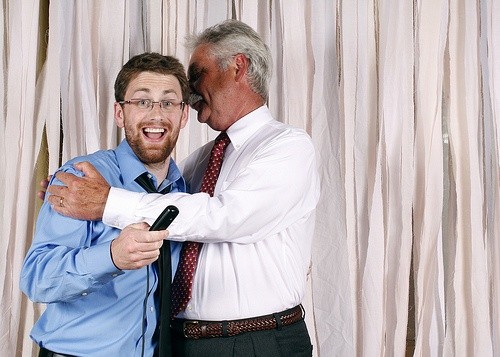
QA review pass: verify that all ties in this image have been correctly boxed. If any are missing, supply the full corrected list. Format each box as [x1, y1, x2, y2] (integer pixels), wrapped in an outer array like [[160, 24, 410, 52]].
[[140, 175, 174, 357], [167, 132, 230, 318]]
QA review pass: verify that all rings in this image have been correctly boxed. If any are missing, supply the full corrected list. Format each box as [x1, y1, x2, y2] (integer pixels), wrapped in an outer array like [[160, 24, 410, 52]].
[[60, 197, 64, 207]]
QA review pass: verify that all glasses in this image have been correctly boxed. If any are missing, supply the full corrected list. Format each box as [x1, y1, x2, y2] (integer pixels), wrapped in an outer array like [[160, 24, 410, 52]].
[[118, 99, 185, 111]]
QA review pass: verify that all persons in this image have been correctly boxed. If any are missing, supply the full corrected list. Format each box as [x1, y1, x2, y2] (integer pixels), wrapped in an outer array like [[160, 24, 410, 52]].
[[38, 20, 320, 357], [19, 52, 189, 357]]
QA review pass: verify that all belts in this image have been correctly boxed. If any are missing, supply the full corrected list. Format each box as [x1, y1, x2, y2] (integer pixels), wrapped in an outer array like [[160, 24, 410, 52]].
[[182, 306, 301, 339], [38, 348, 76, 357]]
